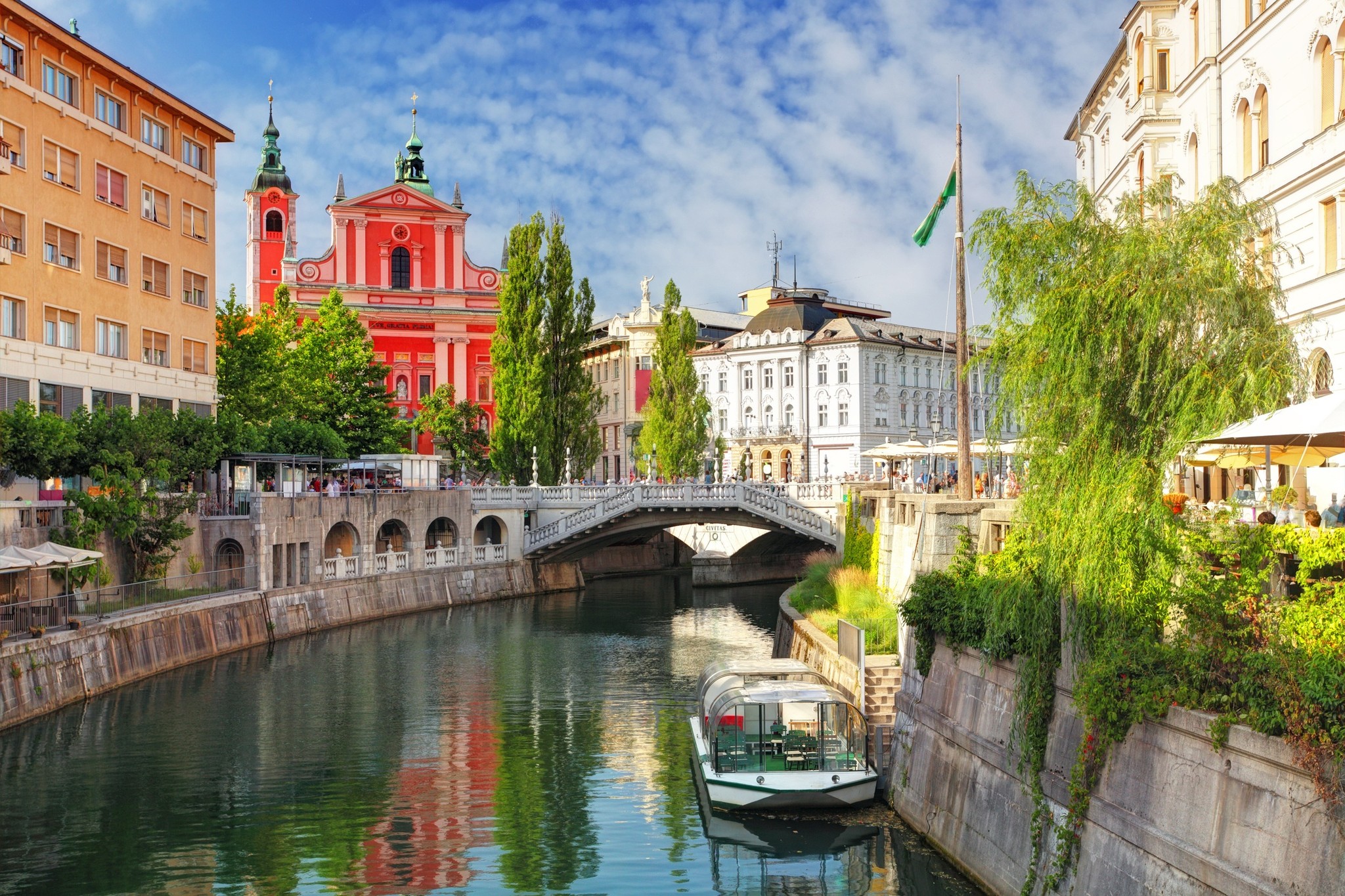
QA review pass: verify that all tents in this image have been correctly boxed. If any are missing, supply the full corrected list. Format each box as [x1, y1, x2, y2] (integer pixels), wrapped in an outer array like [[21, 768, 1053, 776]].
[[1176, 390, 1345, 524], [0, 541, 104, 628], [697, 658, 871, 773]]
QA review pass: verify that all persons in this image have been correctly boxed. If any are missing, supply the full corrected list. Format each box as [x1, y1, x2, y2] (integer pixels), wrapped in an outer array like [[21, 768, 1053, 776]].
[[1336, 506, 1345, 527], [1302, 510, 1321, 528], [892, 471, 897, 490], [916, 470, 958, 494], [266, 478, 275, 492], [440, 476, 484, 490], [704, 470, 714, 484], [1257, 511, 1275, 524], [570, 476, 585, 486], [902, 470, 907, 482], [658, 477, 662, 484], [854, 471, 859, 482], [671, 473, 683, 484], [843, 472, 848, 481], [229, 487, 234, 507], [974, 472, 1021, 500], [640, 477, 646, 485], [691, 476, 696, 484], [307, 472, 402, 497], [730, 468, 739, 481]]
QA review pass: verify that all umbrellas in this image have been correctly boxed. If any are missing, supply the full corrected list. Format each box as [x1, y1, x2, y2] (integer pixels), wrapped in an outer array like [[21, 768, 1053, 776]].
[[859, 436, 1069, 488]]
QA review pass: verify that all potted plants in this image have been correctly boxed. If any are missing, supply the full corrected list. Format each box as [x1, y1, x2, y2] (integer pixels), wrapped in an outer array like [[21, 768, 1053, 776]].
[[30, 627, 47, 639], [69, 618, 82, 629], [0, 630, 9, 647]]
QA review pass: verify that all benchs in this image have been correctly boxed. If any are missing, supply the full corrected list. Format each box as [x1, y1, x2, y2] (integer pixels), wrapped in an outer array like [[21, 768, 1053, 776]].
[[752, 747, 778, 756]]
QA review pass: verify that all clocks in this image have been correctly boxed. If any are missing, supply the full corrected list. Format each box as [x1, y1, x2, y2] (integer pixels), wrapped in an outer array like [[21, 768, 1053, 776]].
[[268, 191, 281, 203], [395, 225, 408, 239]]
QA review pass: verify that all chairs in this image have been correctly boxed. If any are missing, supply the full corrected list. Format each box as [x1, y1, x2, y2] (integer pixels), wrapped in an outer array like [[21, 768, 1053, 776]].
[[712, 724, 752, 773], [770, 722, 858, 771]]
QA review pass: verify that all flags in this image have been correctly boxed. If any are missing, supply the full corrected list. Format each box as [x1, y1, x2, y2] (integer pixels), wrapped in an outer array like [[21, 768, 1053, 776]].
[[912, 157, 956, 249]]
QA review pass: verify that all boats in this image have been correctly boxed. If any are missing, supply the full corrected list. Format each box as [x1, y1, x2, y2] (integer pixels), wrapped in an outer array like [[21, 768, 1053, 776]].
[[690, 655, 878, 814]]
